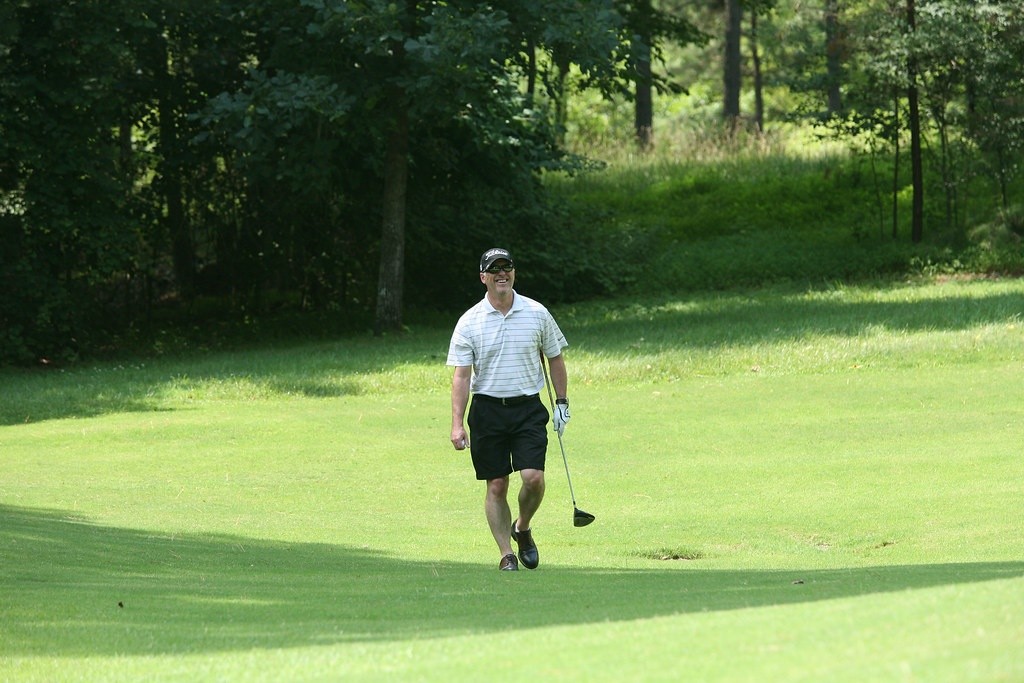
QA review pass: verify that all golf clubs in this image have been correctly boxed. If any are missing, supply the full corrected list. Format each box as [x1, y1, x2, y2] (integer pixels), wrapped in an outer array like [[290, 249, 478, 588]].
[[541, 347, 596, 527]]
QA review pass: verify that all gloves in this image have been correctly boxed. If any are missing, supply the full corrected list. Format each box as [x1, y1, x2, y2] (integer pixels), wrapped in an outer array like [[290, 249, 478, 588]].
[[552, 404, 570, 438]]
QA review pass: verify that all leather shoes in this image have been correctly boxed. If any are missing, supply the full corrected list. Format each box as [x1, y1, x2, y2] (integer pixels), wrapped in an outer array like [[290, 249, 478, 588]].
[[510, 519, 539, 569], [498, 552, 519, 571]]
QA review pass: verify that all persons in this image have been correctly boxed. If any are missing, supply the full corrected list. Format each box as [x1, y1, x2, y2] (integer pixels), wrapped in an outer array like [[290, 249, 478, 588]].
[[446, 248, 569, 571]]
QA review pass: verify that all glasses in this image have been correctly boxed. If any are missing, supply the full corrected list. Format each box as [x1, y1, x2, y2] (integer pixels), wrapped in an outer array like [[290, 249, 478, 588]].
[[485, 263, 515, 274]]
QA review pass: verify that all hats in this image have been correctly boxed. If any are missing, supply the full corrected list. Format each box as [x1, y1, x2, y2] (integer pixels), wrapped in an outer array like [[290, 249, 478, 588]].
[[479, 248, 514, 273]]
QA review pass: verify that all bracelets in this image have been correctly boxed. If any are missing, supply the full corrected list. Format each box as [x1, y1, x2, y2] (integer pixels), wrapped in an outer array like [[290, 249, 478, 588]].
[[555, 398, 569, 405]]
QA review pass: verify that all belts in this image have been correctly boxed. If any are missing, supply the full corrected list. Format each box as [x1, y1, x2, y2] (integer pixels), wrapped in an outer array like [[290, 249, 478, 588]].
[[473, 393, 539, 406]]
